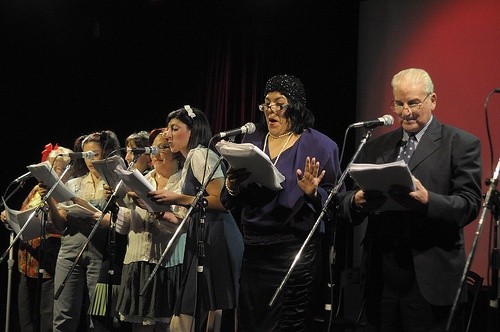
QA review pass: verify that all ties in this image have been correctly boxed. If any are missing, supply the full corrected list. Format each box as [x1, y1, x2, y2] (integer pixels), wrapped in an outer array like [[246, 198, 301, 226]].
[[396, 135, 415, 166]]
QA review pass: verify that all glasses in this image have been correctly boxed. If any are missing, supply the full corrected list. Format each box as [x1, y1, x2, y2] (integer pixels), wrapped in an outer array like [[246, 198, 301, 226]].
[[258, 104, 289, 113], [54, 167, 66, 173], [390, 93, 430, 114]]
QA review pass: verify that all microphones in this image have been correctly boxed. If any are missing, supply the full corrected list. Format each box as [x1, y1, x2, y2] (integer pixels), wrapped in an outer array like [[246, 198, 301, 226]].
[[214, 122, 256, 139], [120, 145, 159, 154], [348, 114, 394, 129], [13, 171, 34, 184], [59, 150, 95, 159]]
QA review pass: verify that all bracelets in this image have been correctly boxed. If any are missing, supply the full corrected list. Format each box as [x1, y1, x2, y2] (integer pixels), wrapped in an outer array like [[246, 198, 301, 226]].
[[155, 211, 165, 219], [225, 177, 238, 196]]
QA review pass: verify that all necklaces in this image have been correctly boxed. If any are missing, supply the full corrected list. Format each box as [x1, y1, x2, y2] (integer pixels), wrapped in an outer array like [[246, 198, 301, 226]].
[[263, 131, 295, 166]]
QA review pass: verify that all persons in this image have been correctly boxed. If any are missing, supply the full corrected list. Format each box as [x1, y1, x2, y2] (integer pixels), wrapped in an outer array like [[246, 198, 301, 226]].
[[221, 74, 346, 332], [38, 130, 121, 332], [0, 147, 73, 332], [335, 69, 481, 332], [152, 105, 244, 332], [88, 127, 187, 332]]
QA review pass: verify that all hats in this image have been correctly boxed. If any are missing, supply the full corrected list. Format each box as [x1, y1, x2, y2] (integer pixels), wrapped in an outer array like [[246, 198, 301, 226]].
[[264, 75, 306, 102]]
